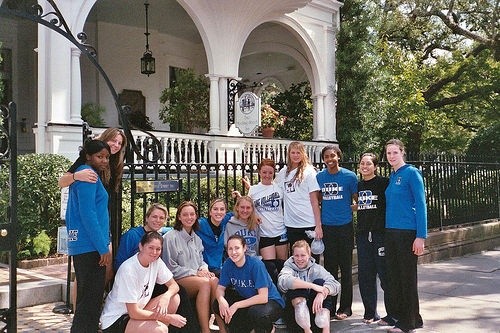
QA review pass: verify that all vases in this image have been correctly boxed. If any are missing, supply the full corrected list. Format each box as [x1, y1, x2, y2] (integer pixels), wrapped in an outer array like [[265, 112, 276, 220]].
[[261, 126, 275, 138]]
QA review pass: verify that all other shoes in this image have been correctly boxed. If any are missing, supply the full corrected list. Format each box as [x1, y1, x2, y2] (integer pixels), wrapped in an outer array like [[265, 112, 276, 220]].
[[387, 327, 416, 333], [378, 320, 389, 326], [336, 313, 347, 320], [364, 318, 374, 323]]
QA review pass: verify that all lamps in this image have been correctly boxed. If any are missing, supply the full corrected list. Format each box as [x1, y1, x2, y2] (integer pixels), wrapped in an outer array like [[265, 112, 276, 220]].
[[141, 3, 155, 77]]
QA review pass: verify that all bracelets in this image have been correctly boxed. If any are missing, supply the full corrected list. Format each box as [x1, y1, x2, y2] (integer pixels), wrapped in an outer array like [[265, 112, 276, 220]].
[[73, 172, 75, 181]]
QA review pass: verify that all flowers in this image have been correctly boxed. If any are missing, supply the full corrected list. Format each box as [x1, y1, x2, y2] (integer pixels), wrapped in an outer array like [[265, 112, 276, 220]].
[[261, 105, 285, 127]]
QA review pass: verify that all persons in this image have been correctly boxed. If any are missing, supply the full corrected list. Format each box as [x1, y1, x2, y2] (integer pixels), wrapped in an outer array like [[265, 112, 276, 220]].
[[98, 139, 429, 333], [58, 128, 128, 324], [66, 139, 113, 333]]
